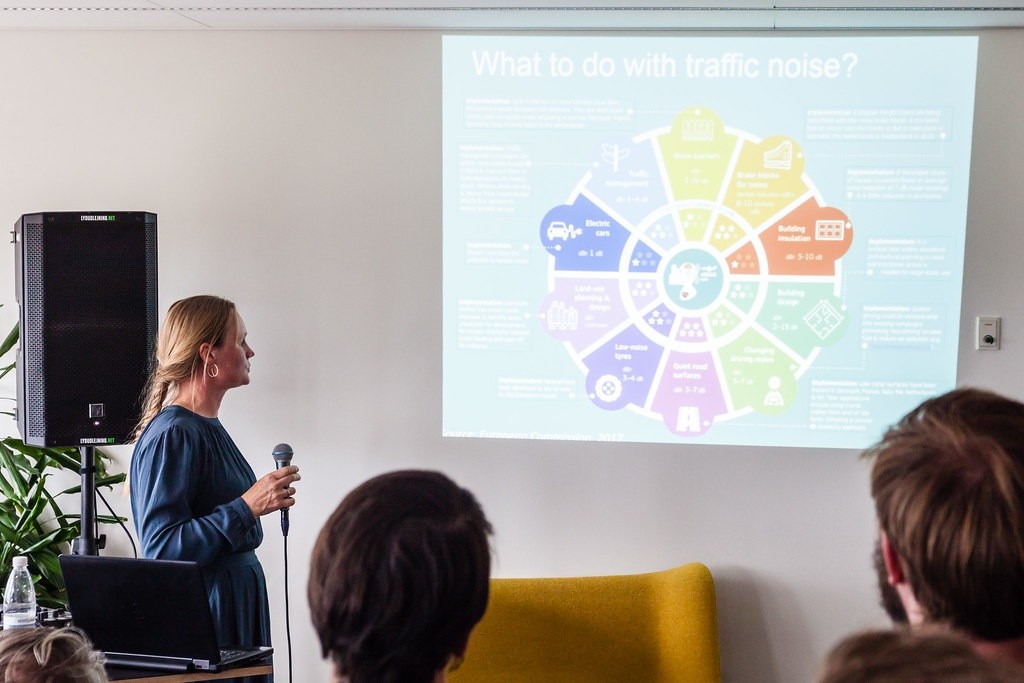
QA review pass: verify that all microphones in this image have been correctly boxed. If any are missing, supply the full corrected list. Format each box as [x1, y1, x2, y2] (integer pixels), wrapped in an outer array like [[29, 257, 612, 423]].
[[272, 443, 294, 537]]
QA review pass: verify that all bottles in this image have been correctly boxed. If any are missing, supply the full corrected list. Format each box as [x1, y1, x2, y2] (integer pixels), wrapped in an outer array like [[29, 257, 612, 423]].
[[3, 557, 37, 629]]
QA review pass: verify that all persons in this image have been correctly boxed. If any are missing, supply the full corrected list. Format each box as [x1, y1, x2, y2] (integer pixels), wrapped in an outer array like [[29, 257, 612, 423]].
[[307, 469, 495, 683], [130, 296, 301, 683], [818, 386, 1024, 682], [0, 628, 107, 683]]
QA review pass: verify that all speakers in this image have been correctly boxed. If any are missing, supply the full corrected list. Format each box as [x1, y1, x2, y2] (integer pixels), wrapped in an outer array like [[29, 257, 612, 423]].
[[10, 211, 160, 448]]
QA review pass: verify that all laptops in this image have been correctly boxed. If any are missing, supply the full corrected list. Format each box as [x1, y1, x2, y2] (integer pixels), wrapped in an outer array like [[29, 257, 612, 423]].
[[59, 554, 275, 673]]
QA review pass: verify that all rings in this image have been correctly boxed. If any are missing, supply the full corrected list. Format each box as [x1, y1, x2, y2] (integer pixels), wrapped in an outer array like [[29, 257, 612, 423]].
[[285, 489, 291, 496]]
